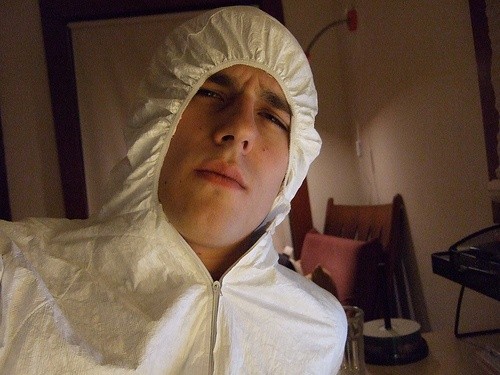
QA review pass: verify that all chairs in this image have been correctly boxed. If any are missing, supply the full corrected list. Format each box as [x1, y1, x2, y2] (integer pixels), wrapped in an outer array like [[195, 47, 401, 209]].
[[279, 193, 403, 325]]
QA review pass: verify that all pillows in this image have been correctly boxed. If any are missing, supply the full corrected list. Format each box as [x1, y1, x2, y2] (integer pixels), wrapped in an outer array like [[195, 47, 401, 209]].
[[279, 227, 381, 315]]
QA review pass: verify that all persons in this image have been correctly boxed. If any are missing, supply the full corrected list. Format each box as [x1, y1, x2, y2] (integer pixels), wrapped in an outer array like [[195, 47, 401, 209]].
[[0, 4, 348, 375]]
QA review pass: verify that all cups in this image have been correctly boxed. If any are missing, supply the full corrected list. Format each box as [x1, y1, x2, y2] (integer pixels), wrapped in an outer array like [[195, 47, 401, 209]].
[[337, 305, 366, 375]]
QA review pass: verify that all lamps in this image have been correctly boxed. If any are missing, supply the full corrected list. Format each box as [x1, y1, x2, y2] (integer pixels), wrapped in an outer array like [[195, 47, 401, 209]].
[[304, 9, 358, 65]]
